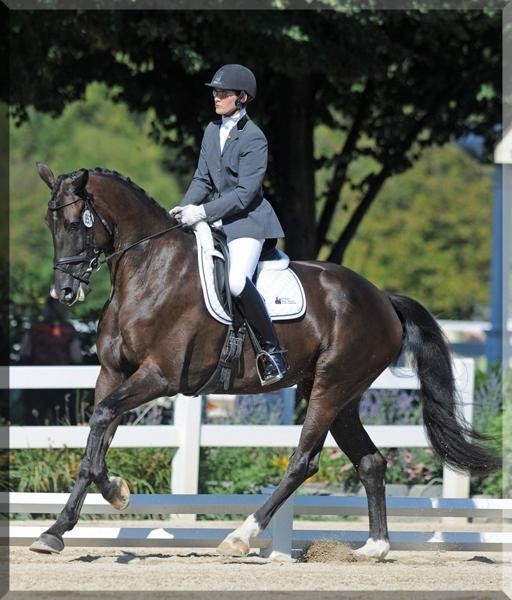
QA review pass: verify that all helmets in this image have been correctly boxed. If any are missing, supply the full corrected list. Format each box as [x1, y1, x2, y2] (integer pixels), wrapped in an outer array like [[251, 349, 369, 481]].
[[205, 65, 257, 97]]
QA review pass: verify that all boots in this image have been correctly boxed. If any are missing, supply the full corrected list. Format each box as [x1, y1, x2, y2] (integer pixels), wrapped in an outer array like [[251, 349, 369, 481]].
[[234, 277, 289, 381]]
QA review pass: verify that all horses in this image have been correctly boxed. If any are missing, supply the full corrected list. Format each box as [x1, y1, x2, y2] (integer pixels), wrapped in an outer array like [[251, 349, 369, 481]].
[[28, 160, 501, 563]]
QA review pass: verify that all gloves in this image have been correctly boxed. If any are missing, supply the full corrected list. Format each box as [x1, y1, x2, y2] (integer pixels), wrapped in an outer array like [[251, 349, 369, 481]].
[[168, 204, 206, 228]]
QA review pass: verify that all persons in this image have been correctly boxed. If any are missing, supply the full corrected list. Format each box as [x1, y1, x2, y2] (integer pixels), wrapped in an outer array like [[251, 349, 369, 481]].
[[168, 64, 291, 381]]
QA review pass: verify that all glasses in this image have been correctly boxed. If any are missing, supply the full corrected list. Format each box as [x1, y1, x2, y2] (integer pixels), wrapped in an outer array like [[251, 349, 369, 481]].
[[212, 89, 234, 97]]
